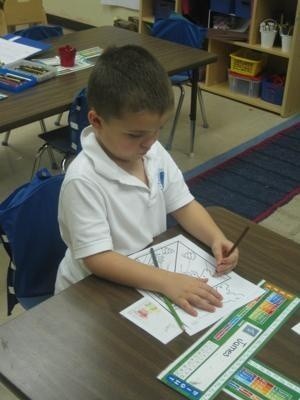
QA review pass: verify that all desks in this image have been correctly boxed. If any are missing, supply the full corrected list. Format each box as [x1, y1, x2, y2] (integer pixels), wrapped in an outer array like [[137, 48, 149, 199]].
[[0, 26, 218, 159], [0, 206, 299, 400]]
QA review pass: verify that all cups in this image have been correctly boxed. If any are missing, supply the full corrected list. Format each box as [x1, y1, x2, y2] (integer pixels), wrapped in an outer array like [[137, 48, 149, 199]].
[[58, 46, 76, 67]]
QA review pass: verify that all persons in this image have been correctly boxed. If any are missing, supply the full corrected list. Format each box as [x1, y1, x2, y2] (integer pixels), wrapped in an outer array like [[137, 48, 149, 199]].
[[53, 42, 241, 316]]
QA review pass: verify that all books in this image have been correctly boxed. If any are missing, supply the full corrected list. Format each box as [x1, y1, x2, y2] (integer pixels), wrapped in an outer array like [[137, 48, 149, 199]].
[[1, 33, 51, 67]]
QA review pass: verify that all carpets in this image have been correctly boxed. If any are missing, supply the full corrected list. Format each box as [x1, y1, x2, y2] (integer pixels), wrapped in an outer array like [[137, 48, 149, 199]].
[[165, 115, 300, 242]]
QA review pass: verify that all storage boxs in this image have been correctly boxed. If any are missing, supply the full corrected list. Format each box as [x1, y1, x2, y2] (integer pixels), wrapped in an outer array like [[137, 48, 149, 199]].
[[228, 69, 261, 98]]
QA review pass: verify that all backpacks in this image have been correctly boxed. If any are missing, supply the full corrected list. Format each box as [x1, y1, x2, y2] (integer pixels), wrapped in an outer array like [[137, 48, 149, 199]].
[[149, 13, 202, 75], [1, 168, 64, 298]]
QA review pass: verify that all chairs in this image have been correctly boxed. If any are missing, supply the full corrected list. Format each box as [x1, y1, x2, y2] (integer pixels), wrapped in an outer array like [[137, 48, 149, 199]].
[[32, 86, 89, 181], [0, 175, 62, 312], [1, 0, 48, 34], [1, 26, 66, 170], [0, 10, 7, 39], [152, 15, 209, 150]]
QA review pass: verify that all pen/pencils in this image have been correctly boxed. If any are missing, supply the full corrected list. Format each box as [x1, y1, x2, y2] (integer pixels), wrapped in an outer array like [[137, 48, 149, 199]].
[[150, 248, 159, 268], [263, 21, 278, 32], [0, 65, 50, 88], [212, 225, 250, 276], [163, 295, 186, 332]]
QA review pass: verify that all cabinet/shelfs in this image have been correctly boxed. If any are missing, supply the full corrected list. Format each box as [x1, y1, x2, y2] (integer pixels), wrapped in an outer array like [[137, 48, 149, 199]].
[[138, 0, 300, 118]]
